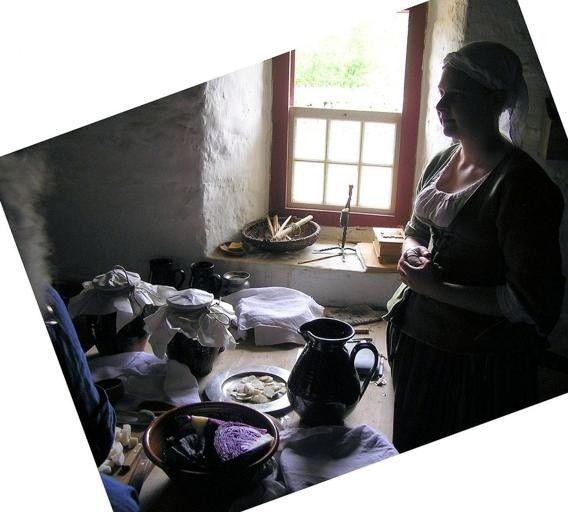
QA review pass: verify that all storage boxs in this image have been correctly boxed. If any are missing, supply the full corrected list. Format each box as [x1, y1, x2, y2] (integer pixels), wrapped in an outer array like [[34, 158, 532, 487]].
[[370, 224, 405, 262]]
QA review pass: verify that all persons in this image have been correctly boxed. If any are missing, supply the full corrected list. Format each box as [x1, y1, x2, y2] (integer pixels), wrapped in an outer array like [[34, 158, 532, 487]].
[[381, 41, 568, 453]]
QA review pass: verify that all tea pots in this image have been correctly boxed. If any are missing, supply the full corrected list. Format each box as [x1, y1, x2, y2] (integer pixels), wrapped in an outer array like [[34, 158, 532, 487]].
[[148, 255, 185, 289], [285, 317, 379, 426], [187, 259, 222, 297]]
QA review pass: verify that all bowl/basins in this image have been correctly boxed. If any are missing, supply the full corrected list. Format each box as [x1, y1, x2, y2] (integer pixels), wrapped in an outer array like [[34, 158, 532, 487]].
[[92, 378, 125, 404], [141, 402, 280, 494]]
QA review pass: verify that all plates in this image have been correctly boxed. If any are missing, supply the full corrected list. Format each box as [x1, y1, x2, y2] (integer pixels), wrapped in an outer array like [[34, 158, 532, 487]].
[[219, 241, 249, 256], [205, 362, 297, 414]]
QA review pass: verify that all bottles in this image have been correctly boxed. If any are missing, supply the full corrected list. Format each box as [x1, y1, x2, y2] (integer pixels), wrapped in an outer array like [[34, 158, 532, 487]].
[[222, 270, 251, 295]]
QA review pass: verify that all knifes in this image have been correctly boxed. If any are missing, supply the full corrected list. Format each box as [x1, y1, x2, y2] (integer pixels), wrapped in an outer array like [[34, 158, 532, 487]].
[[122, 435, 145, 471]]
